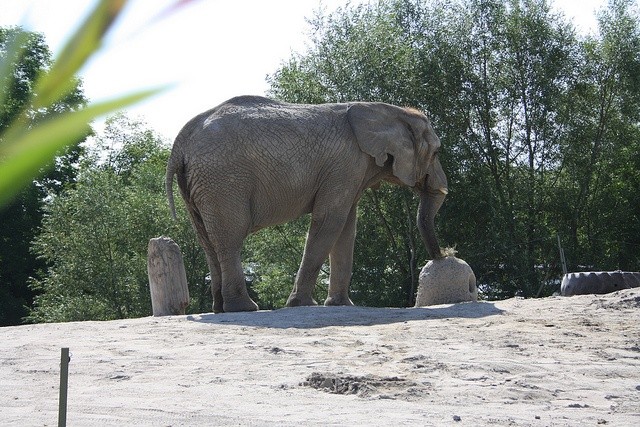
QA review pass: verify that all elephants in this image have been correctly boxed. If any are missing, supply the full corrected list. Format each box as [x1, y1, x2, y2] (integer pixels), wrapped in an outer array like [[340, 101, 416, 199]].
[[166, 96, 448, 313]]
